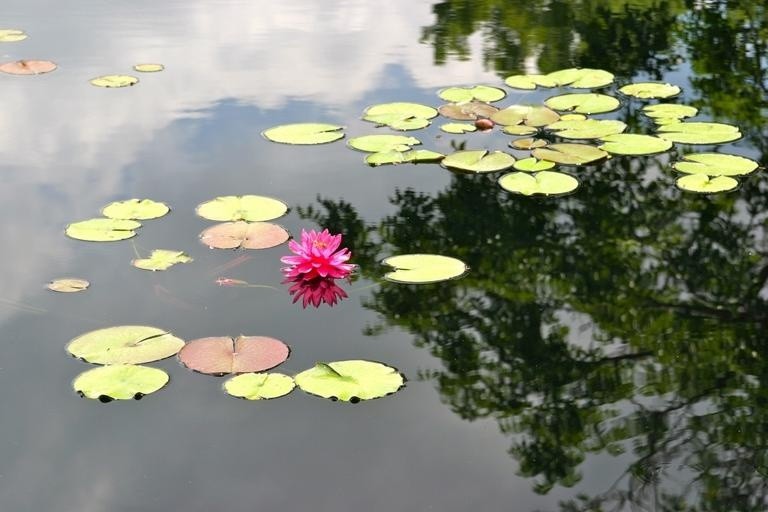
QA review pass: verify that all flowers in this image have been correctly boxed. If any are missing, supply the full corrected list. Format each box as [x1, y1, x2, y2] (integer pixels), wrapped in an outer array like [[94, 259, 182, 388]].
[[280, 228, 358, 282], [288, 283, 349, 309]]
